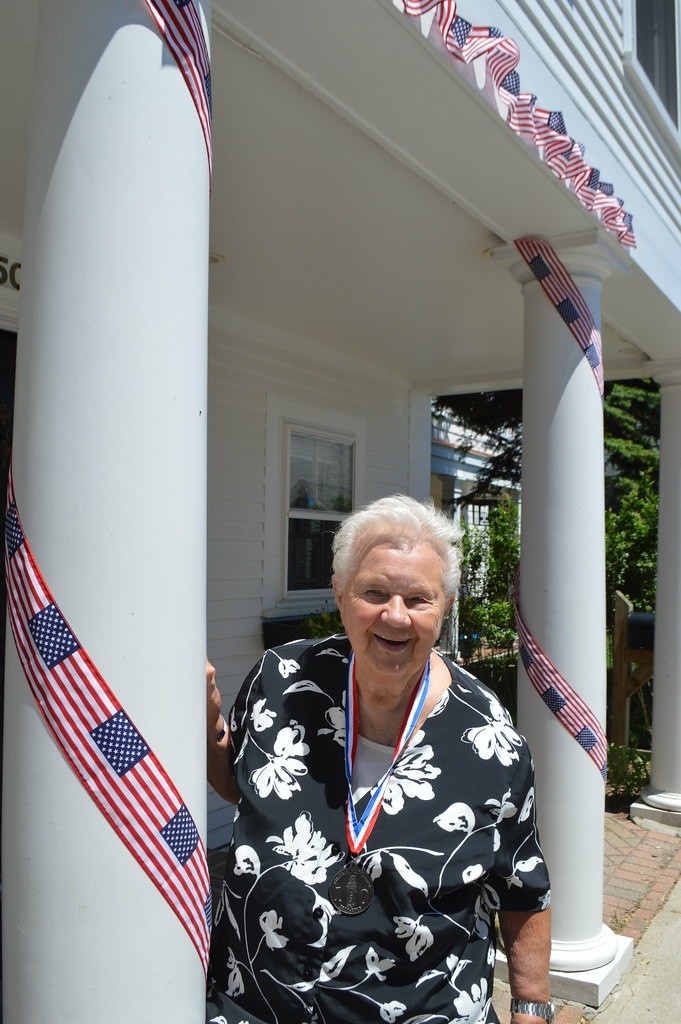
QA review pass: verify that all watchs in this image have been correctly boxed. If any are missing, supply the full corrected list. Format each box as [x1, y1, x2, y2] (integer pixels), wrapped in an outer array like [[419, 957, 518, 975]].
[[510, 998, 555, 1023]]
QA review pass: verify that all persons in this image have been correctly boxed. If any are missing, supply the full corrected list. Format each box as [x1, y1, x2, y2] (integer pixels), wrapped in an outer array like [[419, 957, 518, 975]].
[[206, 493, 554, 1024]]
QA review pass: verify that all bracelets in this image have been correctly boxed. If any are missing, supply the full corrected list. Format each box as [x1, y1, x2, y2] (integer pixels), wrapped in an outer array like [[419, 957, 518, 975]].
[[215, 714, 227, 741]]
